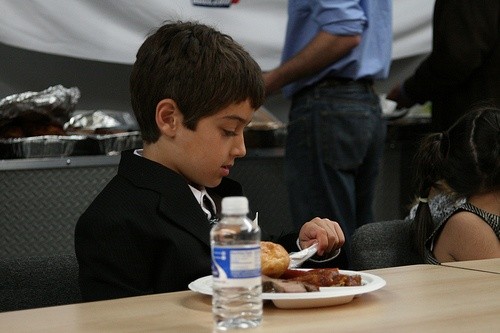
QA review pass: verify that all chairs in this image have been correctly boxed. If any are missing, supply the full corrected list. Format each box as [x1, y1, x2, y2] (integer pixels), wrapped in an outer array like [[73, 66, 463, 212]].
[[0, 249, 80, 312], [350, 217, 418, 270]]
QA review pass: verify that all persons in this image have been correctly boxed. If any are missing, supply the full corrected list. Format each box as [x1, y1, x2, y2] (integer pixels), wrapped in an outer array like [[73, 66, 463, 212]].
[[75, 18, 345, 302], [413, 107, 500, 268], [385, 0, 500, 130], [261, 0, 394, 249]]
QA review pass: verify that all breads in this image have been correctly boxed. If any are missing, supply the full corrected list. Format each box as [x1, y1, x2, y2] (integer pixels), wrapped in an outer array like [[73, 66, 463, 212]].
[[260, 241, 290, 277]]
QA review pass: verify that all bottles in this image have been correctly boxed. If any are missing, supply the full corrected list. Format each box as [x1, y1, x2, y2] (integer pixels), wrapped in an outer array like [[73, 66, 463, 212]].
[[209, 196, 264, 331]]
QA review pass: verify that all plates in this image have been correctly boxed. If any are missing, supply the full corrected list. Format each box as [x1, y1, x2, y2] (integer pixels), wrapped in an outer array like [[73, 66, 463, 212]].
[[188, 268, 387, 310], [384, 109, 409, 125]]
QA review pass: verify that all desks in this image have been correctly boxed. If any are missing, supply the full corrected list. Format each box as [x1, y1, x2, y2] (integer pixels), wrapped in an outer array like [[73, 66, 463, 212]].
[[0, 254, 500, 333]]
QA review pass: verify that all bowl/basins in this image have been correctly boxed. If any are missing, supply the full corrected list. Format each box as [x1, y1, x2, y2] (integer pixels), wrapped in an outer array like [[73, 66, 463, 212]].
[[381, 99, 398, 116]]
